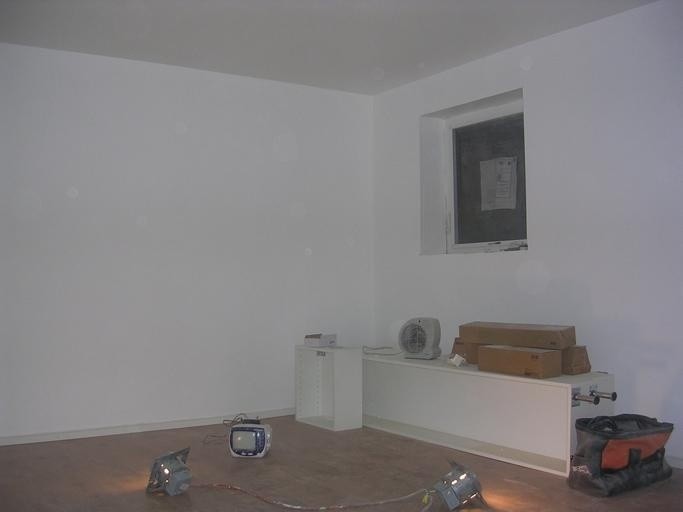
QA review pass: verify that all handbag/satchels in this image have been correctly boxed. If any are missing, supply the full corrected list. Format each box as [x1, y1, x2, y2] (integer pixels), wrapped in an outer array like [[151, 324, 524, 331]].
[[567, 414, 675, 499]]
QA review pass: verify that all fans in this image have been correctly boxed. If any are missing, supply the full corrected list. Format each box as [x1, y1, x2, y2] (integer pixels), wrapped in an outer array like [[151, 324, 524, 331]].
[[394, 315, 442, 361]]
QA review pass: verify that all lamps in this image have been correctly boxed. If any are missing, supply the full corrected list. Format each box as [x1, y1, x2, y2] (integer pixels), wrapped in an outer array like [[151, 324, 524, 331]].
[[144, 445, 196, 497], [427, 457, 491, 511]]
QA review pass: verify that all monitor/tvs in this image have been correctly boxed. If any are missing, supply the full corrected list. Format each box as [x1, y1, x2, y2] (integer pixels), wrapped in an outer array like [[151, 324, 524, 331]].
[[230, 424, 272, 458]]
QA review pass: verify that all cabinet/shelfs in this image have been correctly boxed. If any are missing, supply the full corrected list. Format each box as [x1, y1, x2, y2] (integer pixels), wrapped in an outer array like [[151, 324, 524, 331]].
[[290, 340, 361, 435]]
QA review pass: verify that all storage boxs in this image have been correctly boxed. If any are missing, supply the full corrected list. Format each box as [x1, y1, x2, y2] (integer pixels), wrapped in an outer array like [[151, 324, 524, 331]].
[[447, 319, 592, 381]]
